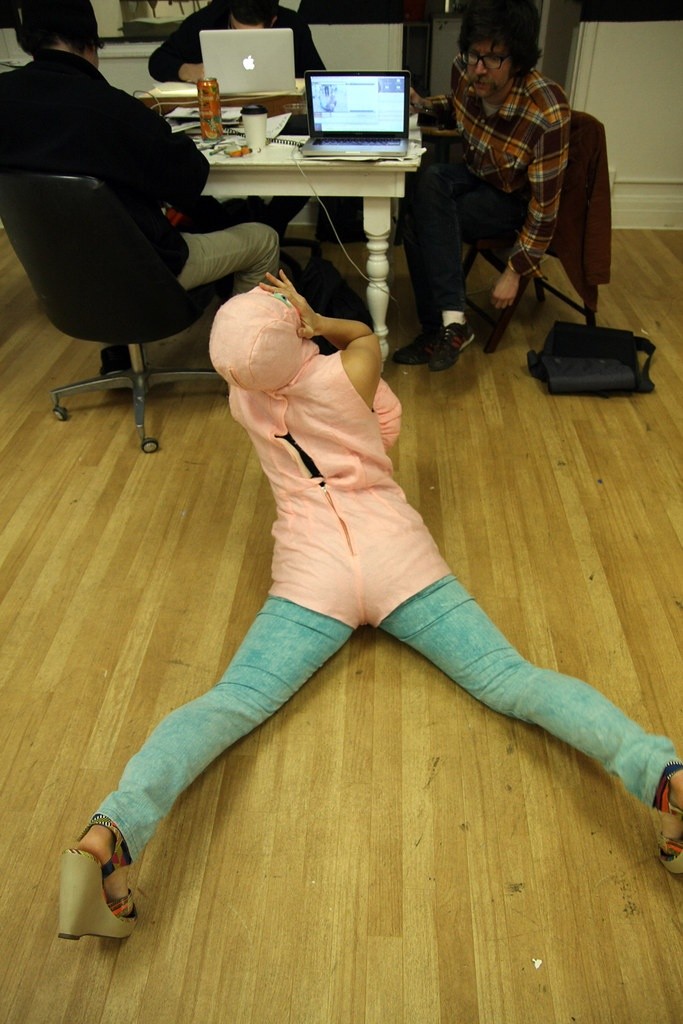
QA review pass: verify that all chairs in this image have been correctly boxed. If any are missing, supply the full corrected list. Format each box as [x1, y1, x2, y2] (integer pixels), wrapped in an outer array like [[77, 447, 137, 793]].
[[453, 110, 609, 355], [0, 175, 230, 453]]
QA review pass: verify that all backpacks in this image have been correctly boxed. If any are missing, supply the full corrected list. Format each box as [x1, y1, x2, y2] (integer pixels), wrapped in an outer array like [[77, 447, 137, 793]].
[[301, 255, 374, 352]]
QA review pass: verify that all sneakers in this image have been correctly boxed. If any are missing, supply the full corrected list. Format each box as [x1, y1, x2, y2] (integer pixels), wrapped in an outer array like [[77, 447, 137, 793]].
[[393, 316, 470, 371]]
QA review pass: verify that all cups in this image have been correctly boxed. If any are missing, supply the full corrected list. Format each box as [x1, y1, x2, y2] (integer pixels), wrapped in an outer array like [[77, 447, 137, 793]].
[[240, 105, 267, 150], [283, 103, 307, 116]]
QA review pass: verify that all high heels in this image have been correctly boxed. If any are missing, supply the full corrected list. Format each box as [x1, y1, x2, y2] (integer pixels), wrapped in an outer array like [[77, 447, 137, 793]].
[[60, 813, 141, 941], [654, 759, 683, 879]]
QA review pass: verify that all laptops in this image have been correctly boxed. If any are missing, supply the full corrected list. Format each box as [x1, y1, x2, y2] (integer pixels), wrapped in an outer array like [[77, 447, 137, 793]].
[[302, 70, 411, 157], [200, 28, 305, 93]]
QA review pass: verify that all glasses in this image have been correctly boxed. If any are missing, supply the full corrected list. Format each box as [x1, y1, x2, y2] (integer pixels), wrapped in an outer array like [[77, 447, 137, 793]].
[[459, 45, 514, 70]]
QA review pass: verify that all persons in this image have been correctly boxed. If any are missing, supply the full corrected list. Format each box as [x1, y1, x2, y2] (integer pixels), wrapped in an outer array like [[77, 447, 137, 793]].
[[0, 0, 279, 297], [148, 0, 326, 241], [391, 0, 571, 371], [57, 272, 683, 942]]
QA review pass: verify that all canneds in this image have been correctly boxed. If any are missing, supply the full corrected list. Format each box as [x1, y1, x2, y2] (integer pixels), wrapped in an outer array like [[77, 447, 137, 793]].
[[197, 78, 223, 140]]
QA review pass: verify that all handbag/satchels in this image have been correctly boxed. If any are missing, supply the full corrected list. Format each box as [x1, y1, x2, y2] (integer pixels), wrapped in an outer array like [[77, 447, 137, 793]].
[[530, 322, 654, 394]]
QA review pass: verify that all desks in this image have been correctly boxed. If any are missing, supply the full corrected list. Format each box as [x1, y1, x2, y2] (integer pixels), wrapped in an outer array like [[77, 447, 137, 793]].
[[136, 111, 424, 375]]
